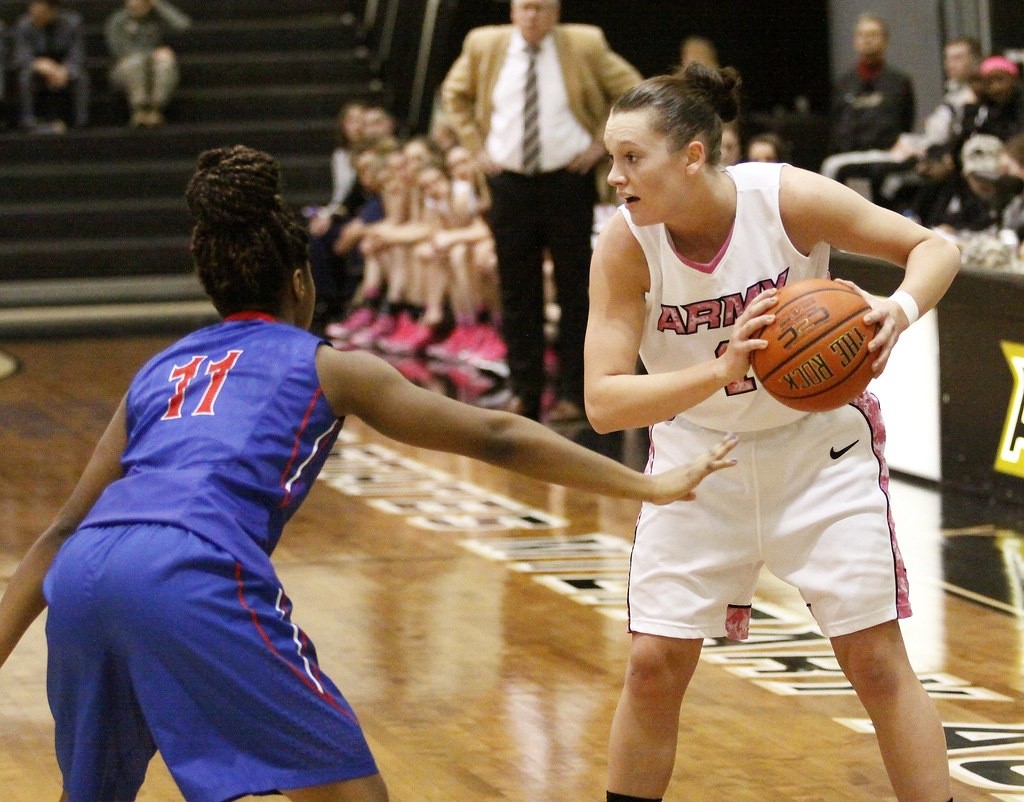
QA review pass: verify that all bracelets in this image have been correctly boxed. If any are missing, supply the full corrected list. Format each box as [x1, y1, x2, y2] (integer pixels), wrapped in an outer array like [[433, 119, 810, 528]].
[[890, 290, 919, 328]]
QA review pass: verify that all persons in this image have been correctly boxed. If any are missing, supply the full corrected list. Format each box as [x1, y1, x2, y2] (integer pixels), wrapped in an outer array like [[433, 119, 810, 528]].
[[10, 0, 93, 133], [103, 0, 192, 127], [582, 62, 961, 802], [0, 144, 740, 802], [307, 105, 624, 382], [442, 0, 647, 416], [682, 16, 1023, 266]]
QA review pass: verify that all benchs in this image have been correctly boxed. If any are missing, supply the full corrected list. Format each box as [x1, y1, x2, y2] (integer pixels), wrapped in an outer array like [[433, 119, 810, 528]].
[[0, 0, 397, 280]]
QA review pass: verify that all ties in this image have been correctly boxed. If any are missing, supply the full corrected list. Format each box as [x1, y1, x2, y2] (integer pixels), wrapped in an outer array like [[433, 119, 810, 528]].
[[521, 46, 542, 175]]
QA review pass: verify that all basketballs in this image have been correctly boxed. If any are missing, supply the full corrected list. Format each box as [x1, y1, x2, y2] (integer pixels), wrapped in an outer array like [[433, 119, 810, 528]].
[[747, 276, 883, 413]]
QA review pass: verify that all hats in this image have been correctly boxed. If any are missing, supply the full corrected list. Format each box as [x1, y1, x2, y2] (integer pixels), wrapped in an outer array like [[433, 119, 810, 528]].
[[961, 134, 1002, 174]]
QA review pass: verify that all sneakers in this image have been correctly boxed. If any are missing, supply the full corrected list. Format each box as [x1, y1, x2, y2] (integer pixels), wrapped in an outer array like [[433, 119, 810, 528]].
[[325, 308, 510, 376]]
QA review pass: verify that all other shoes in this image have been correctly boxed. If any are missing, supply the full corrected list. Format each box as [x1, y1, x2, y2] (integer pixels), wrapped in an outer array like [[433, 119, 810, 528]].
[[512, 395, 540, 423], [132, 110, 162, 126]]
